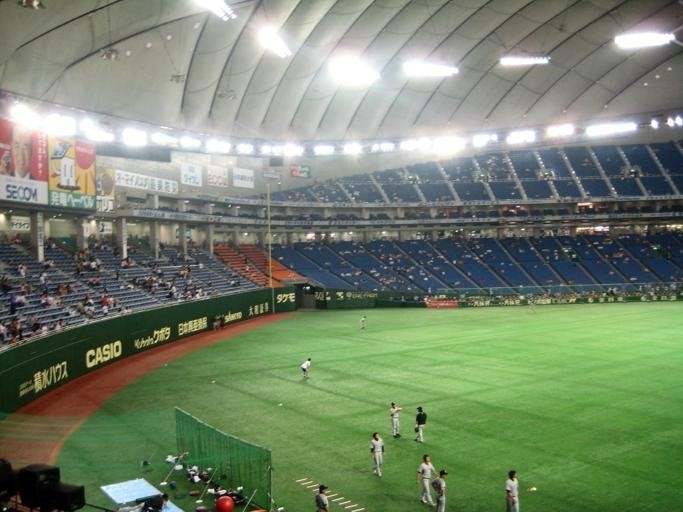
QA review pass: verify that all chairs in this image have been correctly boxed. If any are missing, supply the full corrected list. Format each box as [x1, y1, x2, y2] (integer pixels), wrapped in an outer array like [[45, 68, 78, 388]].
[[268, 233, 681, 304], [404, 143, 680, 229], [2, 236, 267, 345], [118, 194, 266, 220], [269, 167, 422, 204]]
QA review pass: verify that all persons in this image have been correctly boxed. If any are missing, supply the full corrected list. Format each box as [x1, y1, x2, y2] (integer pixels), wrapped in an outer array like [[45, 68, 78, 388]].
[[416, 454, 438, 507], [118, 493, 169, 512], [369, 432, 384, 476], [414, 407, 427, 442], [300, 358, 310, 379], [431, 470, 448, 512], [316, 485, 329, 512], [504, 470, 520, 512], [390, 402, 402, 438]]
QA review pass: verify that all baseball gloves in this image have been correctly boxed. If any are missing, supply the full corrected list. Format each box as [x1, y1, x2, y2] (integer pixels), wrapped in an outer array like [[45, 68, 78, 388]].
[[415, 428, 418, 432]]
